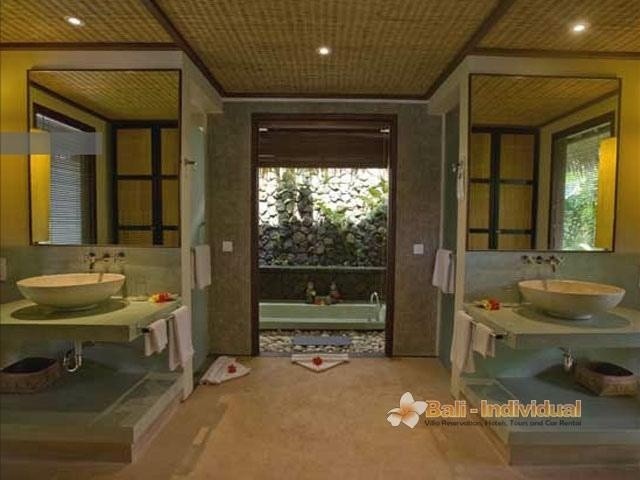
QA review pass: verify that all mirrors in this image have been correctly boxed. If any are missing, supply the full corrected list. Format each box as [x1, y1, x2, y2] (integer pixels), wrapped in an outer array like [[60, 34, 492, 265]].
[[28, 69, 182, 248], [466, 73, 622, 252]]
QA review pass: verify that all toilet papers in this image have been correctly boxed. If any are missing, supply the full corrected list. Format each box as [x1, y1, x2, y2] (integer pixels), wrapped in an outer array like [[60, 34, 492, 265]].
[[329, 283, 340, 302]]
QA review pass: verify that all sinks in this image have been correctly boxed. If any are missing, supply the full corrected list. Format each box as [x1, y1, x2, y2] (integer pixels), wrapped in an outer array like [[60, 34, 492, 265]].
[[16, 273, 125, 311], [517, 279, 627, 320]]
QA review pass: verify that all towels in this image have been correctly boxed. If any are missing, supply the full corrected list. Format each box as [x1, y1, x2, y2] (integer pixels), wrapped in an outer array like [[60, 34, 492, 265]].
[[450, 309, 475, 374], [191, 244, 212, 290], [431, 249, 456, 295], [168, 306, 195, 371], [473, 322, 496, 358], [291, 353, 348, 373], [144, 320, 168, 356], [200, 355, 250, 385]]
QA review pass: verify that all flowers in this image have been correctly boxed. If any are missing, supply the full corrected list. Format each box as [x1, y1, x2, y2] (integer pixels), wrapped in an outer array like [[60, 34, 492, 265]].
[[312, 356, 323, 365], [228, 364, 236, 373], [149, 291, 178, 303]]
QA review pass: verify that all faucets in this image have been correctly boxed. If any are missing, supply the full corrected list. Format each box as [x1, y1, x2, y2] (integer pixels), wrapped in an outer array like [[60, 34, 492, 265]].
[[371, 292, 380, 311], [549, 254, 562, 273], [84, 253, 102, 270]]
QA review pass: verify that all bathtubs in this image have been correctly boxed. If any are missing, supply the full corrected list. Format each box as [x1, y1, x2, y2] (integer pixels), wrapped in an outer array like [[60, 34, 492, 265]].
[[260, 302, 385, 331]]
